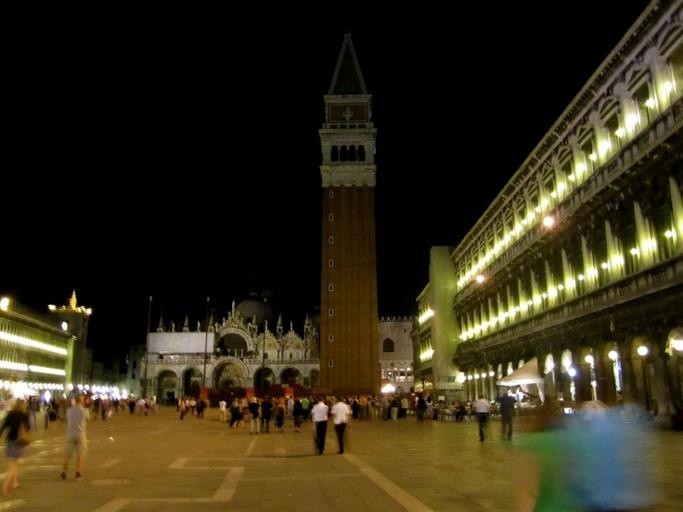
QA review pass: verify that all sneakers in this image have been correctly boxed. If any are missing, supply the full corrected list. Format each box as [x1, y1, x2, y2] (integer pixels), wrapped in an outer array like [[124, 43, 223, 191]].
[[228, 426, 303, 435], [75, 470, 85, 481], [60, 472, 68, 480]]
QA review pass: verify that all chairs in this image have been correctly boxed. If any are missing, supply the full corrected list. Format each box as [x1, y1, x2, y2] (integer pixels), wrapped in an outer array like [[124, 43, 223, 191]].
[[408, 402, 474, 422]]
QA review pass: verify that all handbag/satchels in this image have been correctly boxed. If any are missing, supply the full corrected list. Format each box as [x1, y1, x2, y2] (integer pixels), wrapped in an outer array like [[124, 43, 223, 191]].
[[47, 421, 56, 428], [15, 421, 34, 446]]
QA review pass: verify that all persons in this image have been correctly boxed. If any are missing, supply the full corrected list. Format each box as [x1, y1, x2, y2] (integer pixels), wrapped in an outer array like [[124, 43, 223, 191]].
[[474, 389, 518, 443], [573, 390, 617, 442], [174, 393, 312, 436], [425, 396, 502, 421], [0, 398, 32, 497], [308, 390, 428, 423], [60, 394, 91, 480], [503, 361, 671, 512], [28, 389, 160, 421], [309, 395, 353, 455]]
